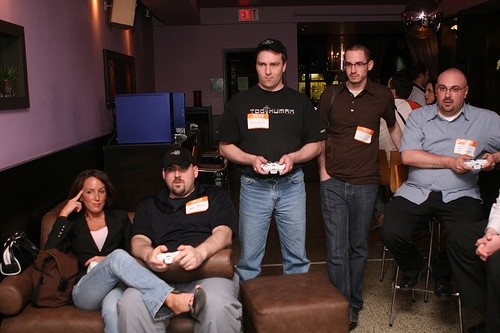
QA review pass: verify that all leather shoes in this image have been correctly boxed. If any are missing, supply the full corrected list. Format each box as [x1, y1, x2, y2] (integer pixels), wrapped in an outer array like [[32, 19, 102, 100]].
[[434, 275, 453, 299], [401, 260, 427, 289]]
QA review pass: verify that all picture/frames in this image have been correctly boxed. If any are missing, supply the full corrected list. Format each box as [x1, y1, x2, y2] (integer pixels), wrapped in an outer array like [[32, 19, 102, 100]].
[[0, 19, 30, 110], [103, 48, 137, 108]]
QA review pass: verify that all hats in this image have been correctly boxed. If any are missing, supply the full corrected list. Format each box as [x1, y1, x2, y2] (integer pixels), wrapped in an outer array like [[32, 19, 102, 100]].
[[162, 146, 193, 171], [256, 38, 288, 60]]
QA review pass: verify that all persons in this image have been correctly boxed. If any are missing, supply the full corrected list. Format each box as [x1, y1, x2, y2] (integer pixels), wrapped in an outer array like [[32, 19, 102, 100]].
[[317, 44, 396, 330], [117, 148, 244, 333], [370, 64, 437, 229], [215, 39, 322, 283], [467, 193, 500, 333], [380, 68, 500, 296], [45, 169, 206, 333]]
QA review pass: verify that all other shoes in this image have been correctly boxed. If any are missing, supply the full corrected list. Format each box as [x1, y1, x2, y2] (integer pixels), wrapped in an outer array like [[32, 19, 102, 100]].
[[369, 214, 385, 230], [467, 322, 487, 333], [375, 240, 387, 247], [348, 305, 359, 332]]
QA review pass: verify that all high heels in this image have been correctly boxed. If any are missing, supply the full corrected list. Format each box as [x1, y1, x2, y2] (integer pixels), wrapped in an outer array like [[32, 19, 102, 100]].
[[175, 287, 207, 323]]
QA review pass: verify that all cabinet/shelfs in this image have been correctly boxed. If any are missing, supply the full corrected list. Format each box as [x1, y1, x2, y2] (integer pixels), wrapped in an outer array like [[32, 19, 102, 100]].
[[103, 106, 212, 213]]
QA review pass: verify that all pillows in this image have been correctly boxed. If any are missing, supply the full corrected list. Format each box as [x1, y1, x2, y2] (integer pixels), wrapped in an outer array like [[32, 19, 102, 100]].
[[133, 247, 234, 283], [0, 263, 36, 316]]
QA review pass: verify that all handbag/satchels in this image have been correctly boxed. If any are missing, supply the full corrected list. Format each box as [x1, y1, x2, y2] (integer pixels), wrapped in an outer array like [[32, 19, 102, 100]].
[[30, 247, 79, 308], [0, 230, 39, 276]]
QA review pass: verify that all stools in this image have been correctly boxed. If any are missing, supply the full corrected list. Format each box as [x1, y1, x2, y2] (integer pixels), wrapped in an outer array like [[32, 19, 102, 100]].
[[377, 148, 464, 333], [240, 270, 350, 333], [196, 142, 229, 189]]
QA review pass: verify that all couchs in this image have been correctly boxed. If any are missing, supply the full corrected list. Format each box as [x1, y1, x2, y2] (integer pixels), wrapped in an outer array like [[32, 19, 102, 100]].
[[0, 199, 235, 333]]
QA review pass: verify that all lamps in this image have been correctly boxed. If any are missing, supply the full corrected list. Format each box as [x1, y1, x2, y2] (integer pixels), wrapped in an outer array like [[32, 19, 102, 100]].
[[403, 0, 442, 39]]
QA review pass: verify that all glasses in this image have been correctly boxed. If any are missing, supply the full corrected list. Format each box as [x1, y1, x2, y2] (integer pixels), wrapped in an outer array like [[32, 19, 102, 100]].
[[437, 86, 467, 93], [344, 60, 371, 67]]
